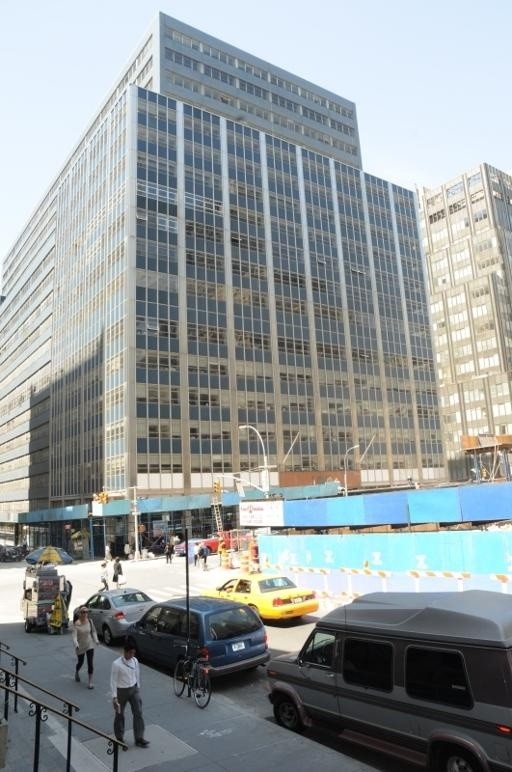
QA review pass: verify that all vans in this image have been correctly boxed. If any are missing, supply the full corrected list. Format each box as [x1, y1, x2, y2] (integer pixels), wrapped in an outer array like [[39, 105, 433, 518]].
[[266, 589, 512, 772]]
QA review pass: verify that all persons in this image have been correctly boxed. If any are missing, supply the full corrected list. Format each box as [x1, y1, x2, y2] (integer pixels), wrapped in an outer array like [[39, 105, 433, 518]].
[[72, 606, 101, 689], [109, 639, 151, 749], [250, 538, 261, 574], [104, 542, 113, 561], [193, 541, 209, 565], [112, 556, 123, 590], [163, 542, 173, 564], [159, 535, 181, 554], [99, 561, 109, 592], [218, 536, 227, 567]]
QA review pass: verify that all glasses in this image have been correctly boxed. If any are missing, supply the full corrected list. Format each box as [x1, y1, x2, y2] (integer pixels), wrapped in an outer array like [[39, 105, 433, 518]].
[[82, 611, 88, 613]]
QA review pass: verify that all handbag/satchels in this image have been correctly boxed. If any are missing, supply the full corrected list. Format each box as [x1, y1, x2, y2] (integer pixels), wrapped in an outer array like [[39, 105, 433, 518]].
[[118, 574, 127, 585]]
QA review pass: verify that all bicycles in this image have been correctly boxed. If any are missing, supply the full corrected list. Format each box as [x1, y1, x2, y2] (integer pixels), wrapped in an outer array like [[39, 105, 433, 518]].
[[171, 639, 214, 710]]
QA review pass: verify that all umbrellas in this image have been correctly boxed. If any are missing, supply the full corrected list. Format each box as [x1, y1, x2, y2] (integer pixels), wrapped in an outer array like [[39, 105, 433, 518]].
[[25, 544, 73, 568], [47, 590, 70, 635]]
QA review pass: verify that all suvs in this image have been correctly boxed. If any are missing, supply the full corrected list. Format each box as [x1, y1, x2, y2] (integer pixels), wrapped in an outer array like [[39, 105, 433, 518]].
[[125, 595, 270, 684]]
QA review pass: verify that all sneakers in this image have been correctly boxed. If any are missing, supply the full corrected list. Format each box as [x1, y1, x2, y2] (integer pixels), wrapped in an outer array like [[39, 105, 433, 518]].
[[89, 685, 94, 689], [75, 673, 80, 682]]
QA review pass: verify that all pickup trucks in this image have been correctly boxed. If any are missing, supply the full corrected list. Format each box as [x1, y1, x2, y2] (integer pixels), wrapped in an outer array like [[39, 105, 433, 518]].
[[194, 531, 249, 558]]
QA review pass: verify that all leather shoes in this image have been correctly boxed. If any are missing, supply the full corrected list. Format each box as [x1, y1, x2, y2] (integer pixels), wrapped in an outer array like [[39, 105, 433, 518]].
[[136, 738, 149, 746]]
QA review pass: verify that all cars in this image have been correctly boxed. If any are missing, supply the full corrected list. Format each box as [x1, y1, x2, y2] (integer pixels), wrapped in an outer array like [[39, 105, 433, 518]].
[[175, 536, 204, 556], [196, 573, 319, 626], [72, 587, 158, 646]]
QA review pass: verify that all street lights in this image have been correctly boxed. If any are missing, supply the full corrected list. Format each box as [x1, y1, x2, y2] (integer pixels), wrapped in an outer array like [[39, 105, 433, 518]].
[[341, 441, 361, 495], [238, 423, 272, 499]]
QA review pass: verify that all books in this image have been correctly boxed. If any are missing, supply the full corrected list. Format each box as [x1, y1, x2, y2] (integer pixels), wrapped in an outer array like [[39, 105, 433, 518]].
[[112, 700, 121, 713]]
